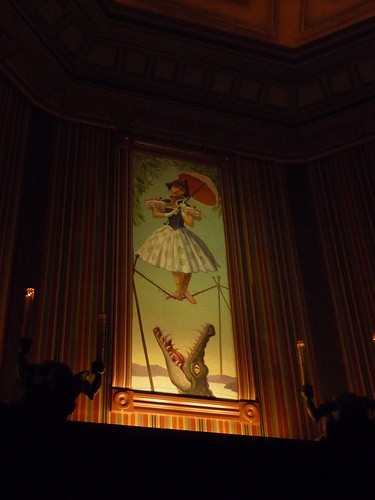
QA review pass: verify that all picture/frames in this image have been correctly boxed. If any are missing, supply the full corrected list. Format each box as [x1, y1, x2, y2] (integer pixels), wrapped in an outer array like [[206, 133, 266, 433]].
[[110, 135, 262, 427]]
